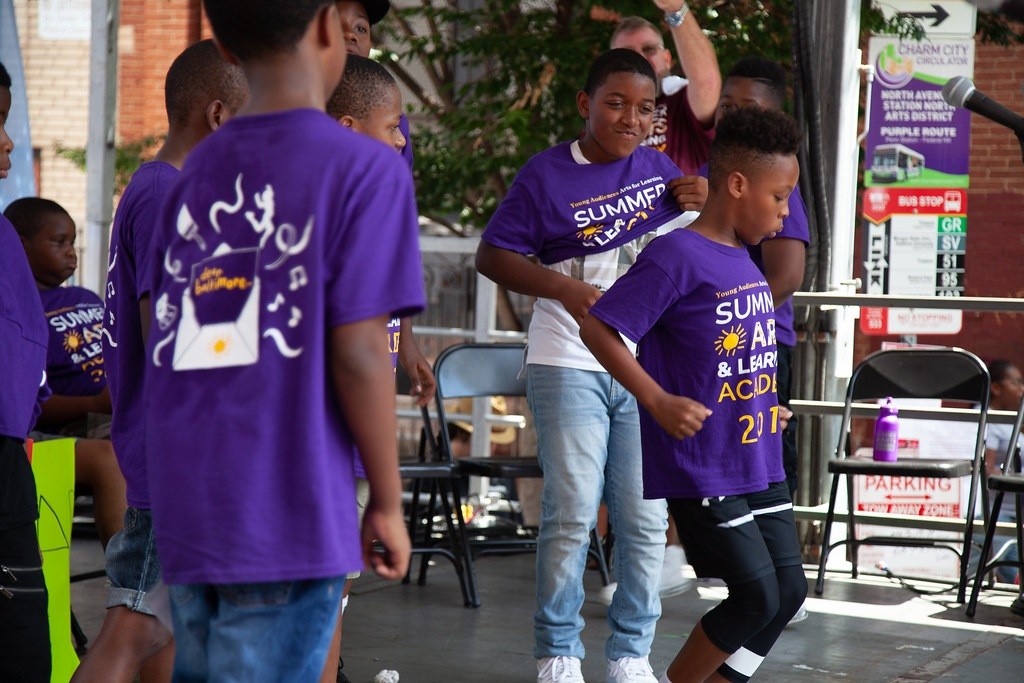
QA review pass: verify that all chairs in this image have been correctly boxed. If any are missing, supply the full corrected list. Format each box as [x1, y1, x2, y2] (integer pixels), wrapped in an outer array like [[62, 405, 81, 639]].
[[355, 362, 472, 608], [815, 347, 997, 605], [418, 342, 611, 608], [966, 392, 1024, 618]]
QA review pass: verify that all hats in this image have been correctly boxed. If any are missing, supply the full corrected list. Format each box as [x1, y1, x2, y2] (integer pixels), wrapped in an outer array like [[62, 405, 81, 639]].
[[356, 0, 390, 27], [451, 395, 516, 445]]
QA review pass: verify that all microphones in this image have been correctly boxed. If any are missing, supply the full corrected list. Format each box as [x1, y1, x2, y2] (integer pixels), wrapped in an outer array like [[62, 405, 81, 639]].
[[942, 76, 1024, 140]]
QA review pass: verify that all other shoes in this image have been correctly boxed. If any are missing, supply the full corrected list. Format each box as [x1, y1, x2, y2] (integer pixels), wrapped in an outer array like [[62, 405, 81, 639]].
[[788, 602, 809, 623], [585, 523, 614, 572]]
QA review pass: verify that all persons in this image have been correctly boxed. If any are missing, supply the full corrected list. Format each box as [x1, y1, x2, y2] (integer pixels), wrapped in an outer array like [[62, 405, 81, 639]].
[[138, 0, 427, 683], [984, 359, 1024, 584], [404, 394, 516, 517], [580, 101, 807, 683], [475, 47, 709, 683], [599, 0, 810, 606], [0, 0, 437, 683]]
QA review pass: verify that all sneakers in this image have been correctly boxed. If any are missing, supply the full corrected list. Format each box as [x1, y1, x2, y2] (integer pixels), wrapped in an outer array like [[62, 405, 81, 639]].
[[536, 655, 585, 683], [605, 654, 658, 682], [598, 545, 697, 604]]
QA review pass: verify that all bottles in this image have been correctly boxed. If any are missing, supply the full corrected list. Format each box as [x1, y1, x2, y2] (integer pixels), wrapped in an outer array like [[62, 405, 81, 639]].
[[872, 396, 899, 462]]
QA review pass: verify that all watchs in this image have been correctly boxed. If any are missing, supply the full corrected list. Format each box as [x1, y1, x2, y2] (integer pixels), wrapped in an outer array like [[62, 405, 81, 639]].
[[664, 1, 689, 26]]
